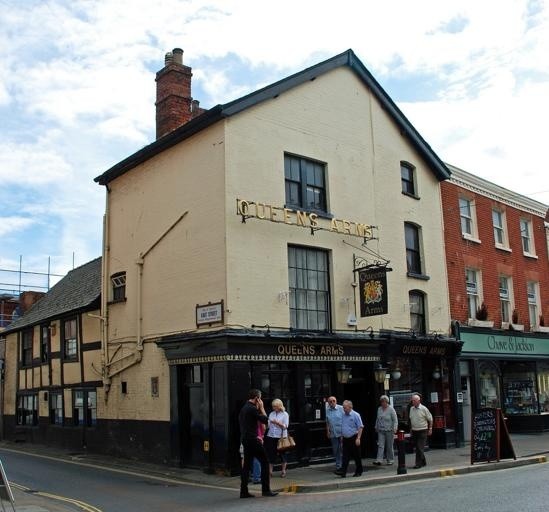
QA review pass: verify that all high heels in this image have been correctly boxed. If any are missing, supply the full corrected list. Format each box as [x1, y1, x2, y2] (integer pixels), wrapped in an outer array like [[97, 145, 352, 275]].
[[281, 470, 287, 478]]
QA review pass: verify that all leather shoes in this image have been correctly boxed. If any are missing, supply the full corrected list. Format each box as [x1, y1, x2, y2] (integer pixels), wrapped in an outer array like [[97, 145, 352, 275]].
[[353, 472, 363, 477], [413, 462, 427, 469], [333, 471, 346, 478], [262, 490, 279, 496], [240, 492, 255, 498]]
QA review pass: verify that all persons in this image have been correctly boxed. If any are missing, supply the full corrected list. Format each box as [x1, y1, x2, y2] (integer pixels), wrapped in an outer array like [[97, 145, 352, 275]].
[[373, 395, 398, 465], [409, 393, 433, 468], [240, 389, 290, 498], [325, 396, 364, 478]]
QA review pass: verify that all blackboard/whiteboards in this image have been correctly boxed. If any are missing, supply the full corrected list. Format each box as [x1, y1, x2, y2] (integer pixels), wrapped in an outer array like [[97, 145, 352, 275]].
[[471, 407, 500, 462]]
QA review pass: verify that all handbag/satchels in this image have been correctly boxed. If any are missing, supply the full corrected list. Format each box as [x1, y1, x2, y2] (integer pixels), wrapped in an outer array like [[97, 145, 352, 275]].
[[277, 428, 296, 450]]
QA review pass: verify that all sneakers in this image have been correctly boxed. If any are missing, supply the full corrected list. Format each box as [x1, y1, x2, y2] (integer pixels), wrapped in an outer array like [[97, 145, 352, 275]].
[[373, 461, 382, 465], [248, 478, 261, 484], [387, 460, 394, 465]]
